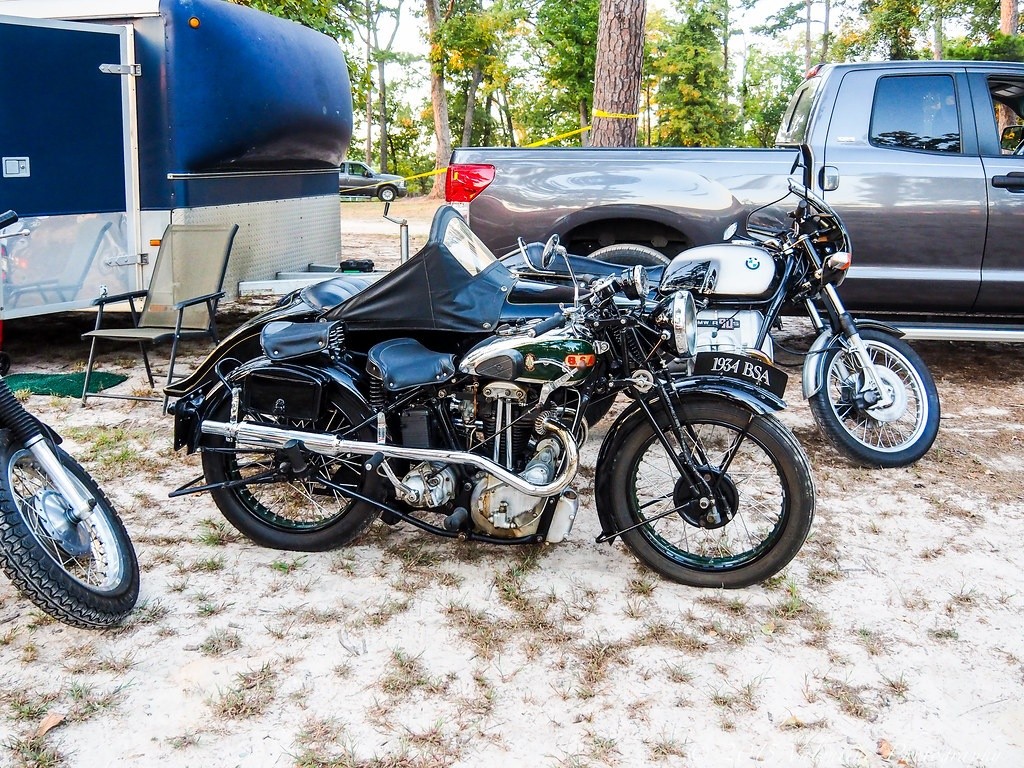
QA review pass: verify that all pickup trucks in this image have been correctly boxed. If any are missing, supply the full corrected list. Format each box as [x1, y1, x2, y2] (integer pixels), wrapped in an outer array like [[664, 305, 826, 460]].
[[448, 46, 1024, 357], [337, 160, 407, 202]]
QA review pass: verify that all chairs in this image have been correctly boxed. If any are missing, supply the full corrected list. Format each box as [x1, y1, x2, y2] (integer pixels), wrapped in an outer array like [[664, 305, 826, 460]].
[[80, 224, 239, 416]]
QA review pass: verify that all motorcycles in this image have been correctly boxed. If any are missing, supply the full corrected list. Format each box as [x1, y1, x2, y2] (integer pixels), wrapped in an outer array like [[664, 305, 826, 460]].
[[437, 146, 943, 476], [168, 222, 821, 595]]
